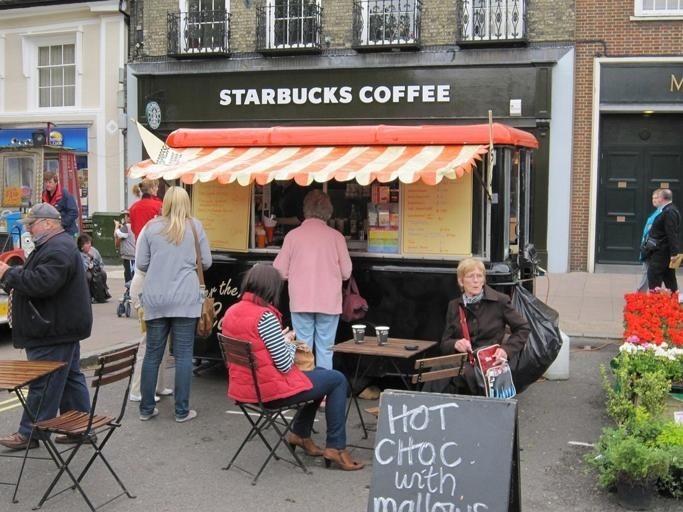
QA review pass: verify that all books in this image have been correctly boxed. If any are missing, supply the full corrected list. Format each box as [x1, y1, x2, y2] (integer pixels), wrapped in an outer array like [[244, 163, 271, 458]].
[[669, 253, 682, 269]]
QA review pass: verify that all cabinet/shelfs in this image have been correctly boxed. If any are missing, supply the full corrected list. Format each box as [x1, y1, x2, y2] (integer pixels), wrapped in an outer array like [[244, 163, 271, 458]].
[[254, 183, 271, 232]]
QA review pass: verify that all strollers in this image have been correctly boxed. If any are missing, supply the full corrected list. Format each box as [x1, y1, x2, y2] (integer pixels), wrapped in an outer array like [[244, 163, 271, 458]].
[[117, 260, 135, 317]]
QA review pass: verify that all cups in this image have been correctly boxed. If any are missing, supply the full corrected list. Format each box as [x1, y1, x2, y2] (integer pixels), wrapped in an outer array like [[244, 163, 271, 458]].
[[374, 326, 389, 346], [351, 325, 366, 344]]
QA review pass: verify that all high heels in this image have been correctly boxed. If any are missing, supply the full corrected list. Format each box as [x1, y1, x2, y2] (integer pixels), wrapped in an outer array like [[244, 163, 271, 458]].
[[288, 430, 325, 457]]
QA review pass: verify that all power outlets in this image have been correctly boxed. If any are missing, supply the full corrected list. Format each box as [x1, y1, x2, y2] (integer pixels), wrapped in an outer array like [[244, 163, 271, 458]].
[[491, 193, 498, 204]]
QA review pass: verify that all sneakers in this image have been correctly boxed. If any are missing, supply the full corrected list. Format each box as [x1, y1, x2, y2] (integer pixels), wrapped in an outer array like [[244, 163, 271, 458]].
[[323, 448, 363, 470], [130, 395, 159, 402], [156, 389, 173, 395], [175, 410, 197, 422], [140, 409, 158, 421], [0, 432, 39, 450], [55, 430, 97, 443]]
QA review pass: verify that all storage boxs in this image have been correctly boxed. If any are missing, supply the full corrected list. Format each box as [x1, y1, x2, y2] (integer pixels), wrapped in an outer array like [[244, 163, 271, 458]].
[[368, 227, 399, 253]]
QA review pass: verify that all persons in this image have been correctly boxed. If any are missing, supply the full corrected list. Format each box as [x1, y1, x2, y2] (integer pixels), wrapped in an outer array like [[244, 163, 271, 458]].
[[134, 187, 212, 422], [130, 264, 174, 402], [634, 190, 661, 292], [41, 172, 80, 235], [0, 201, 96, 447], [272, 189, 353, 410], [75, 235, 107, 286], [422, 257, 528, 396], [641, 188, 682, 294], [222, 263, 364, 470], [114, 220, 137, 281], [128, 177, 163, 237]]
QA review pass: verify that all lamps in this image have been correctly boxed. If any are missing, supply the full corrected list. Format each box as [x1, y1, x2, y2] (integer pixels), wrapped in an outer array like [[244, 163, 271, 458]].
[[11, 137, 19, 144], [22, 140, 29, 145], [31, 129, 46, 146]]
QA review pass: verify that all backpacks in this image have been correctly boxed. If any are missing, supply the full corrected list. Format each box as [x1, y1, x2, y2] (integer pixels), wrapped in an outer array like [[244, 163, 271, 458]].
[[89, 260, 112, 303]]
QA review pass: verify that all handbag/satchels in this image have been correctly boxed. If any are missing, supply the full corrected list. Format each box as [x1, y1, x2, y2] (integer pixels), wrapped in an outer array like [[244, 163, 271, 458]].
[[190, 217, 214, 338], [459, 302, 517, 399], [286, 340, 315, 372], [647, 237, 667, 250], [339, 275, 368, 322]]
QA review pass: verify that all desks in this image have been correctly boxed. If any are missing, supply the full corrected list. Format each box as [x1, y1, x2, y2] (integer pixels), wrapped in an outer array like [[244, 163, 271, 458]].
[[327, 337, 438, 450], [0, 360, 69, 503]]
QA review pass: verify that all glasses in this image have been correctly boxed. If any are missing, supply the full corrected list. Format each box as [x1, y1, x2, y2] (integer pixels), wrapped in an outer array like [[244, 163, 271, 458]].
[[25, 217, 47, 227], [463, 275, 483, 280]]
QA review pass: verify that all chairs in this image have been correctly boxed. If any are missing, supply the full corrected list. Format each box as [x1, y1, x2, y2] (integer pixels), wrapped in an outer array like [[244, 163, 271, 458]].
[[217, 333, 314, 485], [30, 342, 141, 511], [412, 352, 467, 394]]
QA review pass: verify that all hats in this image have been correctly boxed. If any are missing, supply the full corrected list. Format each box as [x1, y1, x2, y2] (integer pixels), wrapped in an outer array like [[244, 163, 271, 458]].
[[20, 202, 61, 224]]
